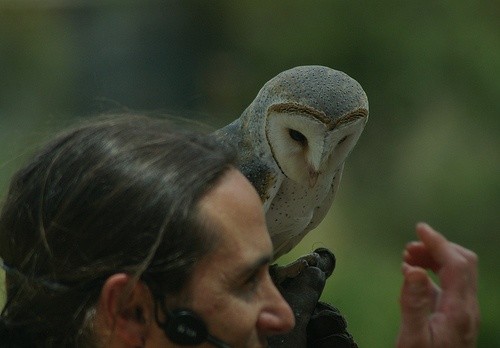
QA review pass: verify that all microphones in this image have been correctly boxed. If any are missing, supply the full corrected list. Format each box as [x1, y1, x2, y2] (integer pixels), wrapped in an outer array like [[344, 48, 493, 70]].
[[164, 306, 232, 348]]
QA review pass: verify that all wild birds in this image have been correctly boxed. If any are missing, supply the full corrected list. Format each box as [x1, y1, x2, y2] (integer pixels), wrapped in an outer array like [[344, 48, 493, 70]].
[[206, 63, 371, 279]]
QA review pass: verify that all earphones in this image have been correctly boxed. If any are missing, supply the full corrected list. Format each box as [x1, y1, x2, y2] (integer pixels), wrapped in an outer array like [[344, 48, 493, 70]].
[[135, 305, 144, 323]]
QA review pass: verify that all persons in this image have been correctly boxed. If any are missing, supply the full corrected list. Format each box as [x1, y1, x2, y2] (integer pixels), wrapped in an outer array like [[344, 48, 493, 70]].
[[1, 117, 479, 348]]
